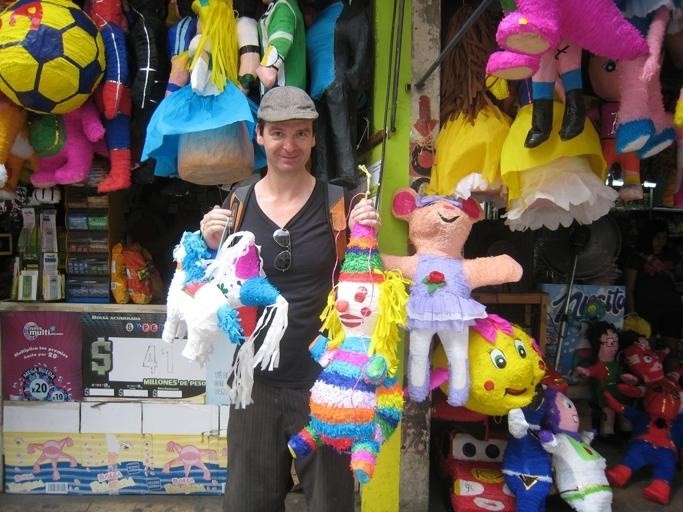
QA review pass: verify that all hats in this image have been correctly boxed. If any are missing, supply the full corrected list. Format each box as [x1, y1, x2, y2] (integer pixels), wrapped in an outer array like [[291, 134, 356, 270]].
[[258, 86, 319, 123]]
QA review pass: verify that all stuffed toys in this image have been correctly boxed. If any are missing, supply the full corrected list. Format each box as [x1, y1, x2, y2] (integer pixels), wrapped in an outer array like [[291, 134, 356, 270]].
[[162, 230, 243, 362], [196, 230, 289, 409], [429, 1, 683, 233], [428, 313, 683, 511], [286, 162, 415, 484], [429, 312, 682, 512], [1, 0, 376, 191], [379, 185, 523, 407]]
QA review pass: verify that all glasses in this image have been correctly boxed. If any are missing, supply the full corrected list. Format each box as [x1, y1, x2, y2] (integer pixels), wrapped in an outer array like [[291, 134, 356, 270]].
[[274, 227, 292, 272]]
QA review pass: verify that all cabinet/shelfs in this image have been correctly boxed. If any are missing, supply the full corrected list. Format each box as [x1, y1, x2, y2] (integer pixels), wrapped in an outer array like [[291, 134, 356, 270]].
[[65, 189, 129, 304], [429, 291, 549, 479]]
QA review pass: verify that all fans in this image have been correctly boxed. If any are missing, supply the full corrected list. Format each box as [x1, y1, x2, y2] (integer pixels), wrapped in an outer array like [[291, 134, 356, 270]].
[[537, 214, 621, 282]]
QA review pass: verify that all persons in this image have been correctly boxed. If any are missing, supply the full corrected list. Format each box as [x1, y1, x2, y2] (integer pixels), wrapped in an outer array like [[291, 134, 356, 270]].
[[625, 219, 682, 338], [426, 0, 683, 232], [1, 1, 376, 192], [200, 86, 382, 512]]
